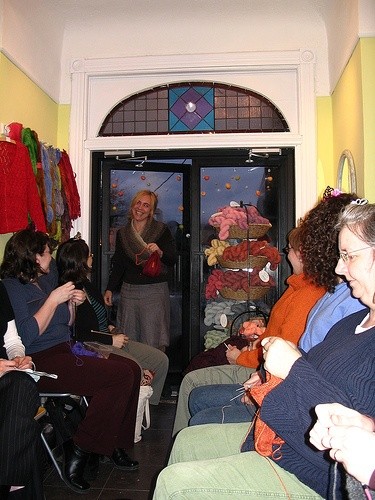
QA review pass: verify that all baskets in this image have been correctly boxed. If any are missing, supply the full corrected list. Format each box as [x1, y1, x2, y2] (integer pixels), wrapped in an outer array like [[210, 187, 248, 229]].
[[217, 287, 271, 301], [214, 223, 272, 240], [215, 254, 269, 269]]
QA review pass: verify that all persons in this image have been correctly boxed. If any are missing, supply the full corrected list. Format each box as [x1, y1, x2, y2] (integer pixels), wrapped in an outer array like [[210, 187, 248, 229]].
[[102, 190, 178, 354], [0, 230, 170, 500], [151, 192, 375, 500]]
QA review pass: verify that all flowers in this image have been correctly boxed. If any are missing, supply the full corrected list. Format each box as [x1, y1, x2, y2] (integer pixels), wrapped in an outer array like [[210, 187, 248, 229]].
[[330, 188, 347, 196]]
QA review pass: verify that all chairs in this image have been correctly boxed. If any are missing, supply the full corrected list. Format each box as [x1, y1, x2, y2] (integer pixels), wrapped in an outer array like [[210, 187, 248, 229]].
[[33, 393, 89, 481]]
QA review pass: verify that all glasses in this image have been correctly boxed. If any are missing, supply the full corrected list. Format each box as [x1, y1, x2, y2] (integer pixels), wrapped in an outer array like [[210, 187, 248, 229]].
[[89, 254, 94, 258], [285, 247, 292, 252], [339, 247, 372, 264]]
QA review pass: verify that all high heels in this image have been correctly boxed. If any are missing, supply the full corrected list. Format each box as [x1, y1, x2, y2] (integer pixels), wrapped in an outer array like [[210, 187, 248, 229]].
[[54, 438, 91, 494], [98, 448, 140, 471]]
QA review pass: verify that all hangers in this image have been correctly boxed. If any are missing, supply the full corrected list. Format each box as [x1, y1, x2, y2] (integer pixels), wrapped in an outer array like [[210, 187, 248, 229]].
[[0, 125, 16, 145]]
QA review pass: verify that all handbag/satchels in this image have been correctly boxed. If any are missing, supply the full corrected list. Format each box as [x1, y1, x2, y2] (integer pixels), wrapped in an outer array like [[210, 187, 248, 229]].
[[133, 385, 153, 444]]
